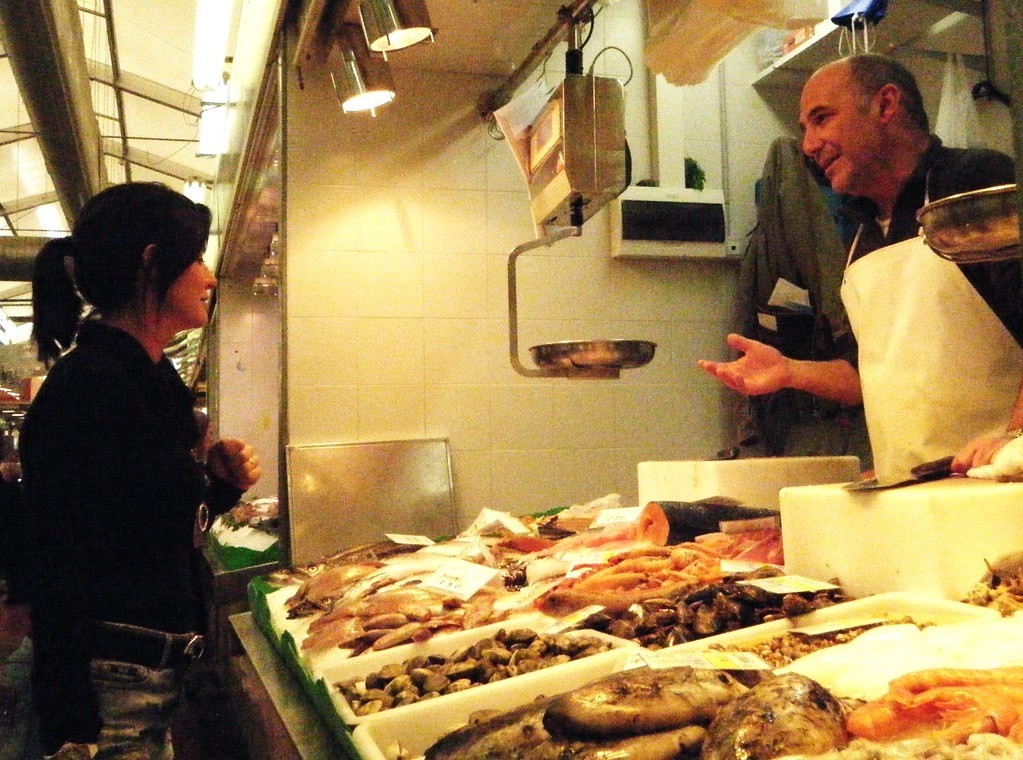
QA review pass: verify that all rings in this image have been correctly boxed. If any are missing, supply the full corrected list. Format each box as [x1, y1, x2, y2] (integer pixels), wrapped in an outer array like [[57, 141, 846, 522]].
[[248, 460, 257, 469]]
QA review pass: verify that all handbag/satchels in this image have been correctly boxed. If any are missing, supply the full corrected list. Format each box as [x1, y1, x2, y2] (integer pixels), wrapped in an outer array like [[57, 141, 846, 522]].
[[935, 54, 985, 151], [831, 0, 888, 59]]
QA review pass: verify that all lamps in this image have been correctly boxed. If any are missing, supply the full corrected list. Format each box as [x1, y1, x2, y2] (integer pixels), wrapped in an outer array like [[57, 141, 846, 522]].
[[357, 0, 432, 59], [328, 22, 397, 118]]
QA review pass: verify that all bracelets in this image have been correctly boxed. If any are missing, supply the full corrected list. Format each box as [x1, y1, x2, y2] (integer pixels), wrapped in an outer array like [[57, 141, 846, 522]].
[[1005, 430, 1023, 439]]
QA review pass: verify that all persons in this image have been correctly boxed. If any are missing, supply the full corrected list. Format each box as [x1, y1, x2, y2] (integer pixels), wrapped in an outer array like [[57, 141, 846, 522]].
[[0, 447, 23, 606], [696, 53, 1023, 476], [17, 182, 263, 760]]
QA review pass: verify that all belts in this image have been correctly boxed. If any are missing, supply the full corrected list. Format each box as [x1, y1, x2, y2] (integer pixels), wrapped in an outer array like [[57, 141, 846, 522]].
[[81, 617, 206, 673]]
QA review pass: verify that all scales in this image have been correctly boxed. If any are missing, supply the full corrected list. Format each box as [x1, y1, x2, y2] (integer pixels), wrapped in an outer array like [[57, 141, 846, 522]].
[[914, 0, 1023, 265], [507, 73, 658, 384]]
